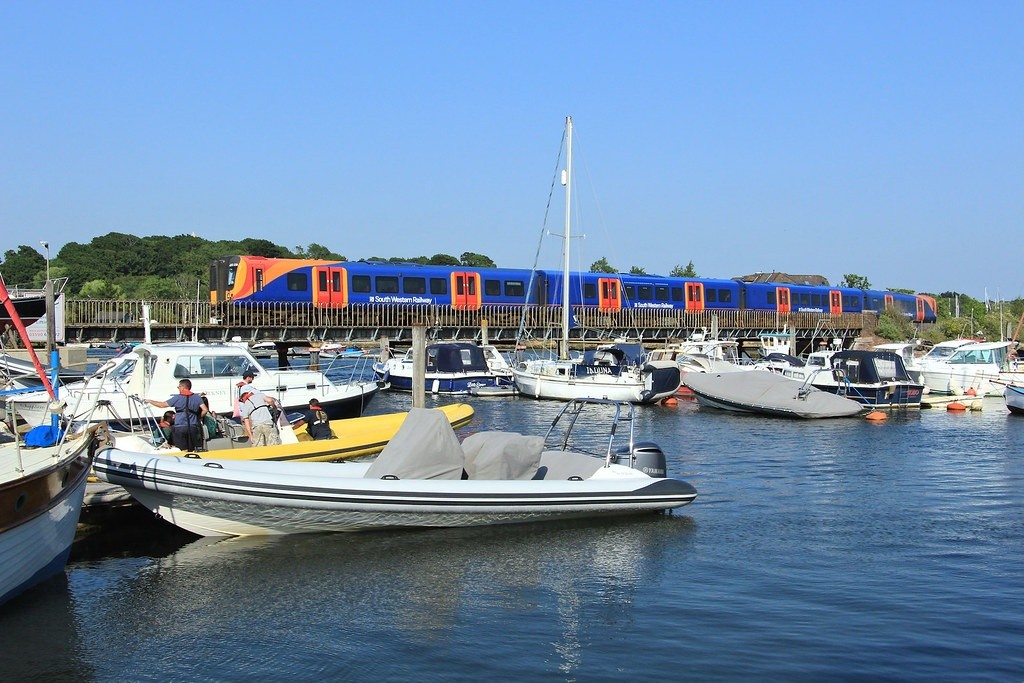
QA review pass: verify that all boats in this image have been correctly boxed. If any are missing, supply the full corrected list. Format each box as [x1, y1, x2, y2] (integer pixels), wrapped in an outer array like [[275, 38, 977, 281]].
[[678, 321, 1024, 419], [7, 277, 383, 433], [82, 403, 477, 485], [1, 274, 99, 592], [110, 320, 701, 544], [372, 339, 520, 397]]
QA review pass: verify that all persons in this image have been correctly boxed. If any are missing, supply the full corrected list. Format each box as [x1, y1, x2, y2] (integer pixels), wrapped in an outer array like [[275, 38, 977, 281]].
[[142, 379, 208, 451], [240, 384, 282, 448], [292, 398, 333, 441], [153, 397, 217, 449], [232, 370, 258, 425]]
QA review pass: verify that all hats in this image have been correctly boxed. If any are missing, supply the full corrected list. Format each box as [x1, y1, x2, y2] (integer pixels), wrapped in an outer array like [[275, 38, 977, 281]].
[[242, 371, 257, 378]]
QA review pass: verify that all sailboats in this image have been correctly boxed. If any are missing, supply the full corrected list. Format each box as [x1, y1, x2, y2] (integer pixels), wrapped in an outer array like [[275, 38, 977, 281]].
[[510, 116, 683, 405]]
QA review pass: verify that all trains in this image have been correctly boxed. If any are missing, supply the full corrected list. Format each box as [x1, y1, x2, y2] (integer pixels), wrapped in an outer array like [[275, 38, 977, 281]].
[[205, 252, 939, 329]]
